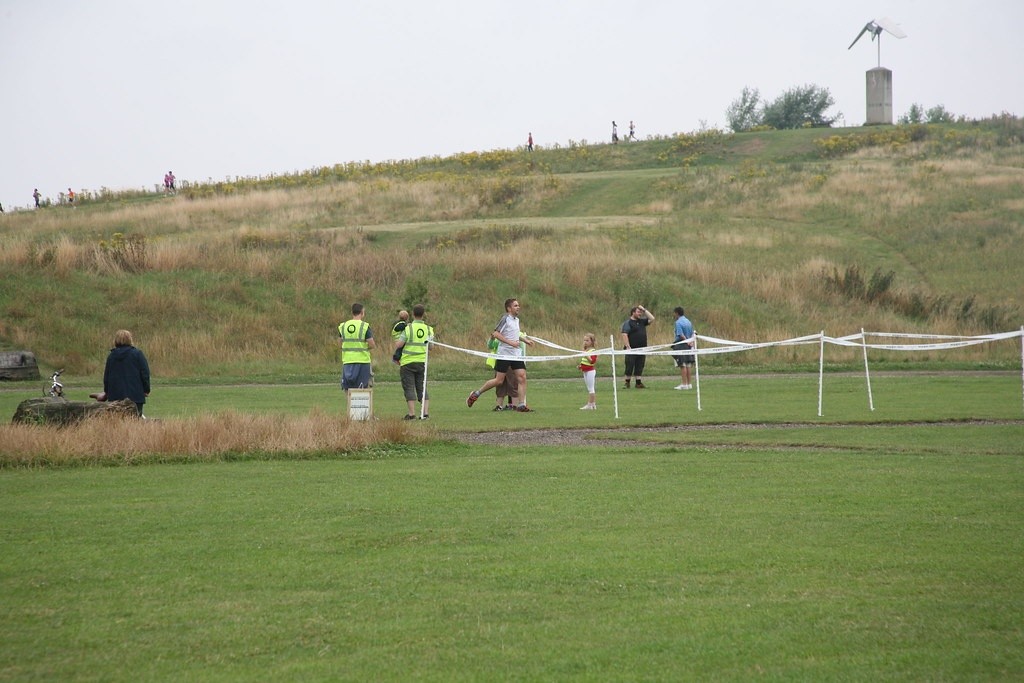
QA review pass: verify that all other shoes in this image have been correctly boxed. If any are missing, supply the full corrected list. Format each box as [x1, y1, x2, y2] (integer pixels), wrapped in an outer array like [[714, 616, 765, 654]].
[[420, 415, 429, 420], [505, 405, 516, 409], [392, 359, 400, 366], [495, 406, 502, 410], [401, 416, 416, 420]]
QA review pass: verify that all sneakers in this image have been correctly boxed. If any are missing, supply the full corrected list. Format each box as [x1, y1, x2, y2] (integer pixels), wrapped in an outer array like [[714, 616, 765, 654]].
[[518, 406, 535, 412], [674, 385, 688, 390], [466, 391, 478, 407], [688, 384, 692, 390], [579, 405, 596, 410], [636, 383, 645, 388], [622, 382, 630, 388]]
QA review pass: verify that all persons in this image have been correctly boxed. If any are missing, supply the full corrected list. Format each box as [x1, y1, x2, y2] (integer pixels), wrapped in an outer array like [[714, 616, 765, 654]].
[[526, 133, 534, 152], [103, 331, 151, 417], [33, 188, 41, 209], [629, 121, 639, 141], [68, 188, 76, 208], [392, 311, 409, 366], [621, 305, 654, 388], [577, 334, 597, 409], [338, 304, 376, 392], [466, 298, 535, 412], [612, 121, 618, 143], [672, 308, 696, 389], [394, 305, 434, 419], [488, 333, 534, 411], [165, 171, 176, 192]]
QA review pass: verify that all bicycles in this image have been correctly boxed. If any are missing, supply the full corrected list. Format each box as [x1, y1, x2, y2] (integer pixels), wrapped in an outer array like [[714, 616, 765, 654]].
[[46, 369, 147, 420]]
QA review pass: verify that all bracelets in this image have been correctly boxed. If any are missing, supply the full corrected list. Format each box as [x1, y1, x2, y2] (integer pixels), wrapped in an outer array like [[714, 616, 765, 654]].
[[643, 308, 647, 312]]
